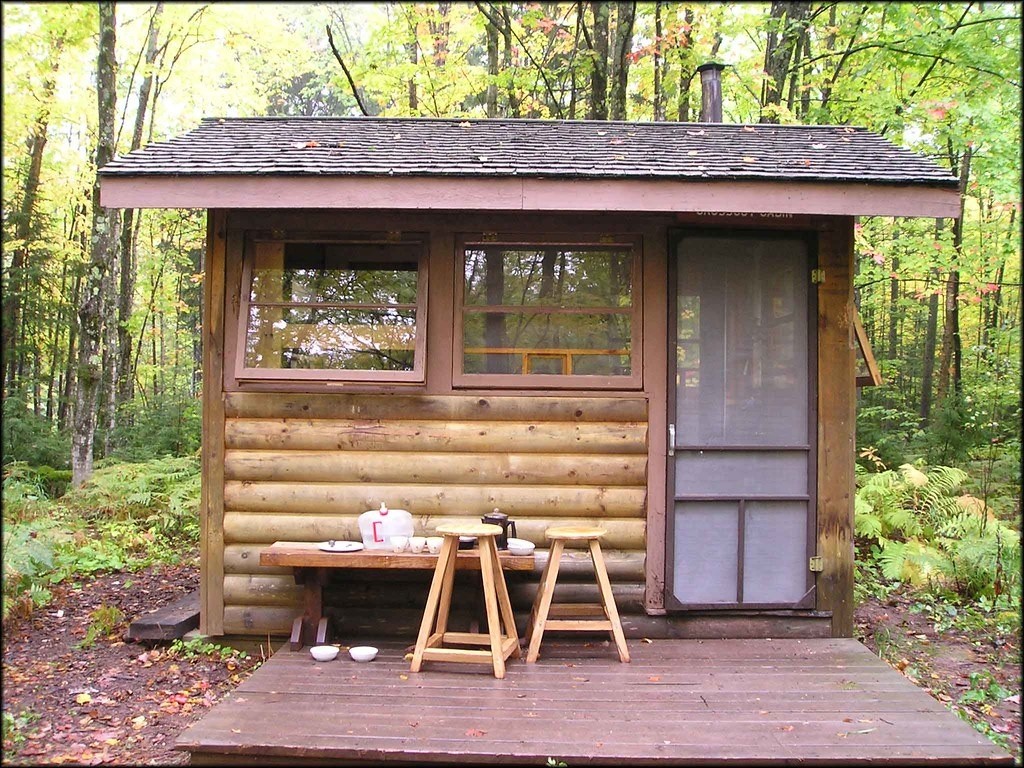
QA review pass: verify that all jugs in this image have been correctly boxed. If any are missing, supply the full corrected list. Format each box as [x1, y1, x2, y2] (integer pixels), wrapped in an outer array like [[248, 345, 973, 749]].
[[481, 508, 517, 551]]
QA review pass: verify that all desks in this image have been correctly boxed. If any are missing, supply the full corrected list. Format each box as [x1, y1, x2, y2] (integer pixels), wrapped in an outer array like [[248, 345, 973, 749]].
[[259, 541, 534, 652]]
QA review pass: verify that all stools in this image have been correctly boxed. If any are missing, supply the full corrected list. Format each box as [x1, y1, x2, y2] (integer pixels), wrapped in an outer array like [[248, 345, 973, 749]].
[[526, 527, 631, 663], [411, 524, 523, 679]]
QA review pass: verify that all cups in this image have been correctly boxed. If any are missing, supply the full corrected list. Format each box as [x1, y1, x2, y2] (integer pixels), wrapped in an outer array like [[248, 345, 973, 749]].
[[409, 537, 425, 553], [427, 537, 443, 554], [389, 536, 407, 553]]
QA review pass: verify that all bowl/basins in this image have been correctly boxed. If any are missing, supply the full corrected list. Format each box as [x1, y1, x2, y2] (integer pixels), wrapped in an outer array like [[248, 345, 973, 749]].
[[309, 645, 340, 661], [349, 646, 379, 662], [507, 537, 535, 556]]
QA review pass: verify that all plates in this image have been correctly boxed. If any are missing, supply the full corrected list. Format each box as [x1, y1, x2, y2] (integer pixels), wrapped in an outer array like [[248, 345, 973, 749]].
[[318, 540, 364, 552]]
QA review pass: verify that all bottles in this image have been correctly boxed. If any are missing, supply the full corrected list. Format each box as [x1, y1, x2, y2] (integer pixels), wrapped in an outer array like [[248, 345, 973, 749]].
[[357, 502, 415, 550]]
